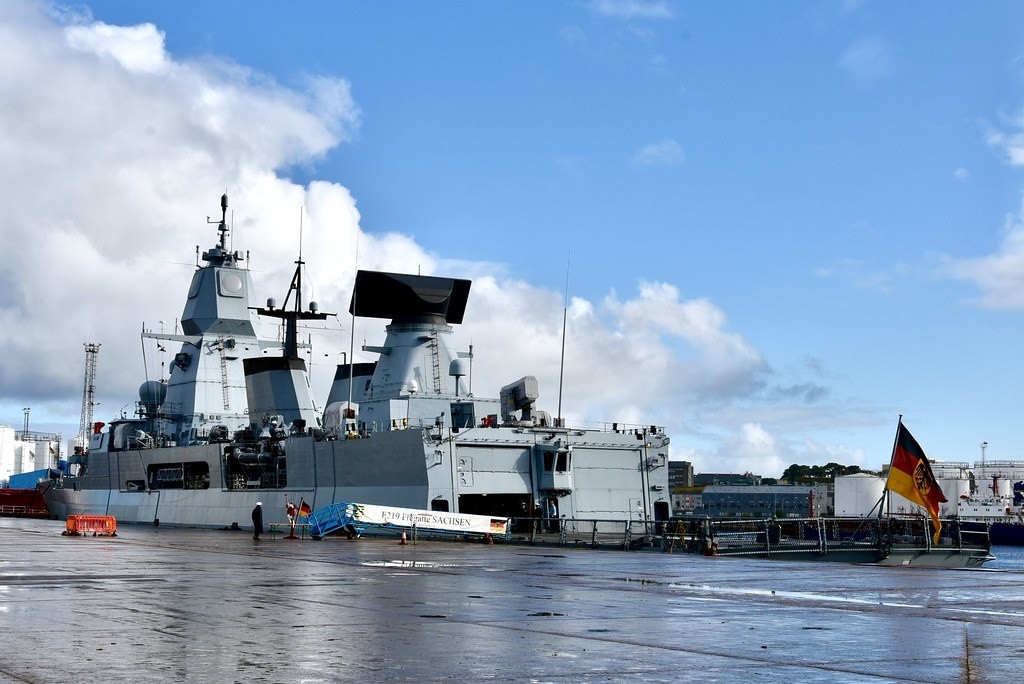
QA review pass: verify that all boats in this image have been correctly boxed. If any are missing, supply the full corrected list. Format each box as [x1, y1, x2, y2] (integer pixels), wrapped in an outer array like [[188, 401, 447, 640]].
[[950, 440, 1024, 542], [33, 185, 675, 538]]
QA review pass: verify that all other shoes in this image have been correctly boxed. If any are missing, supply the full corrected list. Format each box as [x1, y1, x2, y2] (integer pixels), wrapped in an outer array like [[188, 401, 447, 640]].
[[256, 537, 260, 540], [253, 537, 256, 540]]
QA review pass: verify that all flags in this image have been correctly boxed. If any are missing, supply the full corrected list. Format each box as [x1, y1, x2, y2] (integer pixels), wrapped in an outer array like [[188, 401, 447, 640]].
[[298, 501, 311, 517], [49, 447, 56, 455], [885, 421, 948, 546]]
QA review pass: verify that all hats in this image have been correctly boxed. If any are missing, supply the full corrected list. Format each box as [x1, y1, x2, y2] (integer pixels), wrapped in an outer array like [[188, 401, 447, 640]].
[[256, 501, 263, 505]]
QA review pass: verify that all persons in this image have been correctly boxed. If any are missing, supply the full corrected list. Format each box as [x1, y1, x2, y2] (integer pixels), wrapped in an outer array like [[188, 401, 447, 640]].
[[251, 502, 264, 541], [549, 501, 560, 533], [519, 501, 530, 533], [533, 504, 543, 534]]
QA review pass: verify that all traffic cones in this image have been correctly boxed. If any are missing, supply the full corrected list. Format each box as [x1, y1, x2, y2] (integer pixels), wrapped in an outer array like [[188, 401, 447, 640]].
[[398, 528, 408, 545]]
